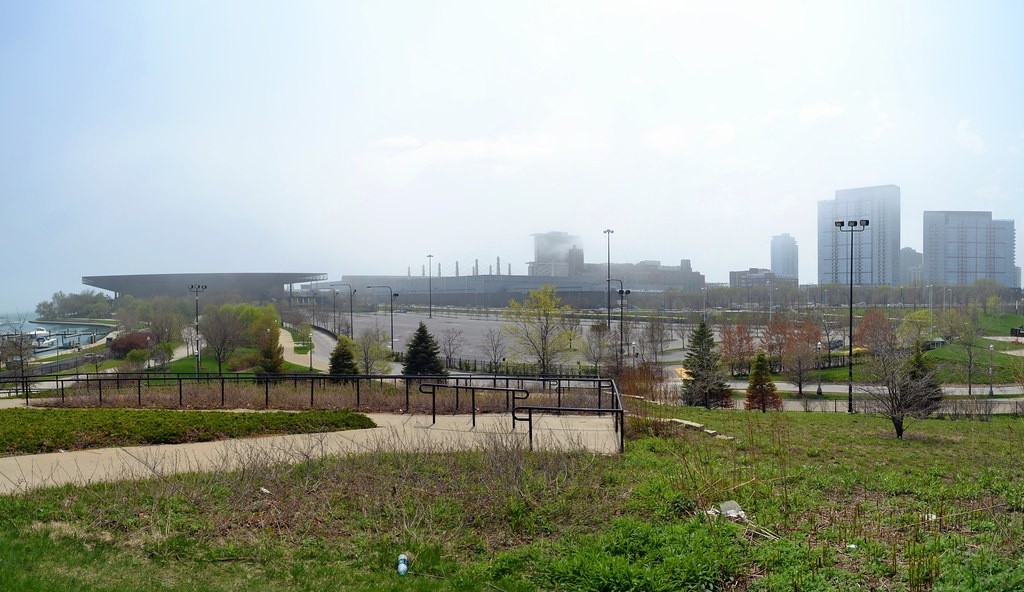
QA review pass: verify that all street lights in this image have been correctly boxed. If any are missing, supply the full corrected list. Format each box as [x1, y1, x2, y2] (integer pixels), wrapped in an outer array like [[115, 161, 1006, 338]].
[[604, 229, 631, 372], [426, 255, 434, 319], [922, 283, 953, 336], [314, 282, 398, 351], [834, 219, 869, 411], [187, 285, 208, 382]]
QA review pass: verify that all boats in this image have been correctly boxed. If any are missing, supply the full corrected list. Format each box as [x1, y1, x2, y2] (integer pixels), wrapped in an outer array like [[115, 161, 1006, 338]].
[[27, 327, 56, 348]]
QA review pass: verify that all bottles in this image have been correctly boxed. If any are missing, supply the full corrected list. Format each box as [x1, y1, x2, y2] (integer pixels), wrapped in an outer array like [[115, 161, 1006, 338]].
[[398, 554, 407, 576]]
